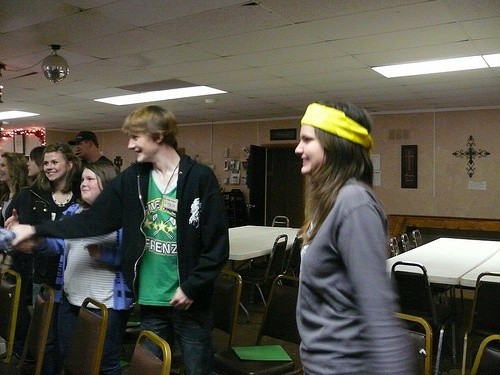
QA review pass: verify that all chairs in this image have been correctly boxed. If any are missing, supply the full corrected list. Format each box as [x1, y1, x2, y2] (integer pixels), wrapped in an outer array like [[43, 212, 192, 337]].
[[0, 284, 55, 375], [387, 228, 500, 375], [128, 330, 172, 375], [211, 215, 304, 375], [0, 270, 21, 362], [60, 297, 108, 375]]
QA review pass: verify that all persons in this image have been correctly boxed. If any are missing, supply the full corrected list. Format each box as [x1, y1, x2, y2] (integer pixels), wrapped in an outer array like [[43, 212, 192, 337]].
[[10, 106, 230, 375], [295, 98, 415, 375], [5, 162, 132, 375], [0, 131, 121, 362]]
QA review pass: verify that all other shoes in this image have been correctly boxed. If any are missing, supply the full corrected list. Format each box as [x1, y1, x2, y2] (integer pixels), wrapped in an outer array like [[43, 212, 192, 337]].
[[12, 355, 20, 366]]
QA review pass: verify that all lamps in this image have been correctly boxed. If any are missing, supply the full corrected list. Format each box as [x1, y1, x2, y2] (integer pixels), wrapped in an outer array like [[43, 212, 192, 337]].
[[42, 45, 70, 82]]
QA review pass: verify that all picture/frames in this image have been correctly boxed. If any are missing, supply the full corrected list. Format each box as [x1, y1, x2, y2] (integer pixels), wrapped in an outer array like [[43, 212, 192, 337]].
[[270, 128, 297, 140], [401, 145, 417, 188]]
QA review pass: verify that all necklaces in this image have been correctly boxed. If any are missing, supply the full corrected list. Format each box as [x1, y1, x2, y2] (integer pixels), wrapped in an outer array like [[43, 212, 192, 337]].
[[151, 164, 178, 209]]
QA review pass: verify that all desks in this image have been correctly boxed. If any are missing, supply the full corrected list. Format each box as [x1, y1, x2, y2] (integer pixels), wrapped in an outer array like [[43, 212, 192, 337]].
[[228, 229, 301, 273], [387, 238, 499, 285], [460, 250, 499, 287], [229, 225, 292, 239]]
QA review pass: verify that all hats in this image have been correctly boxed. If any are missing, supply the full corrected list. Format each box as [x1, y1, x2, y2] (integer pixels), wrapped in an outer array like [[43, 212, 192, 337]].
[[67, 131, 97, 145]]
